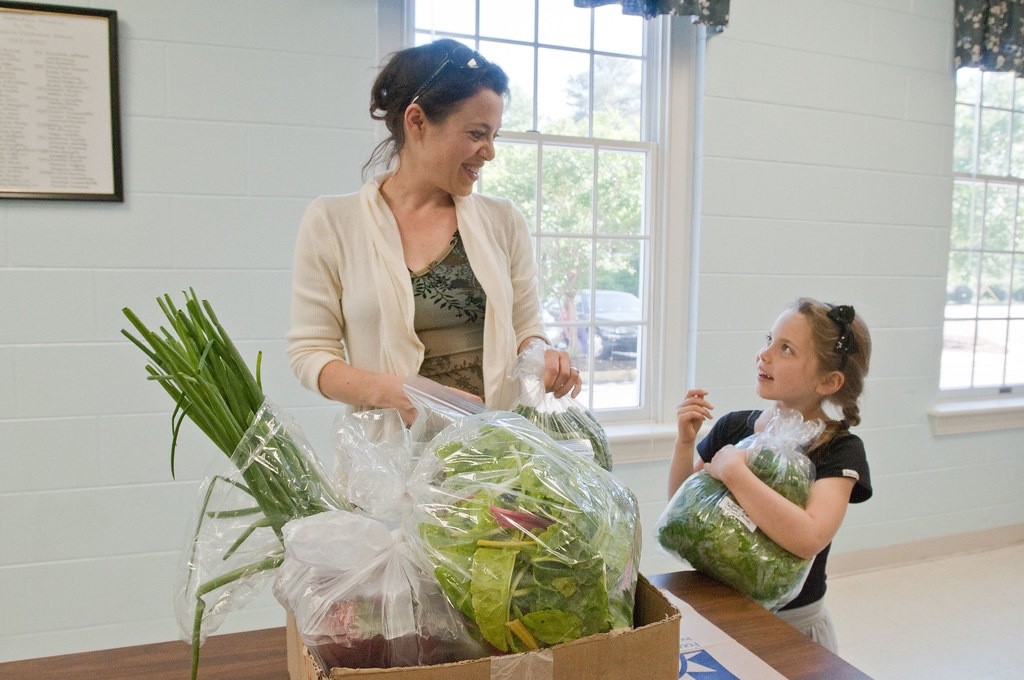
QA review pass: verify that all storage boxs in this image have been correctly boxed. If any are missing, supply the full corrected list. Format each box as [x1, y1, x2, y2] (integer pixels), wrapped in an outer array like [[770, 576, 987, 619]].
[[286, 569, 681, 680]]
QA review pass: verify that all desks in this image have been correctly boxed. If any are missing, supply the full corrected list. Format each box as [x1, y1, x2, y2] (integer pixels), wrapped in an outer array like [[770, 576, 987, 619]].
[[0, 569, 871, 680]]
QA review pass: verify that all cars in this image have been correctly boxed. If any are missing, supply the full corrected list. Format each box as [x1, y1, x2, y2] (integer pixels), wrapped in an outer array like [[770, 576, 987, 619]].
[[561, 289, 639, 360], [538, 306, 573, 355]]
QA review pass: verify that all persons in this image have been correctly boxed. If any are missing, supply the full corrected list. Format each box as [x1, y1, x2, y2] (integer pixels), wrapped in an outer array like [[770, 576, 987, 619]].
[[668, 300, 873, 652], [286, 40, 583, 482]]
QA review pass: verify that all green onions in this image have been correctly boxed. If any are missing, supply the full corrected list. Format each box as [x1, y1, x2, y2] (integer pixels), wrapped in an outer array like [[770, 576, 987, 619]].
[[119, 285, 332, 680]]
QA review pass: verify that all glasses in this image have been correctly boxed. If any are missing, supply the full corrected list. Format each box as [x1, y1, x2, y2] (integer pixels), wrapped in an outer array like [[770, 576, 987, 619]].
[[410, 44, 488, 105]]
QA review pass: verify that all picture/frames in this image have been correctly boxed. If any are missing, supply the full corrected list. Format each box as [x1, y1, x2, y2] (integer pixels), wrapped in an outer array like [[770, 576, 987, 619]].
[[0, 1, 123, 202]]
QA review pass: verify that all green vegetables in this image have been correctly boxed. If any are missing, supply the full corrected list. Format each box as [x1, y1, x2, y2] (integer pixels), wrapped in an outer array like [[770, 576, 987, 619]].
[[657, 447, 814, 603], [411, 398, 642, 654]]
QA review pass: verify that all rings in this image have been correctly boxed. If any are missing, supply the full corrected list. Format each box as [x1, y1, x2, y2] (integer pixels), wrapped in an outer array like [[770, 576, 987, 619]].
[[571, 367, 579, 373]]
